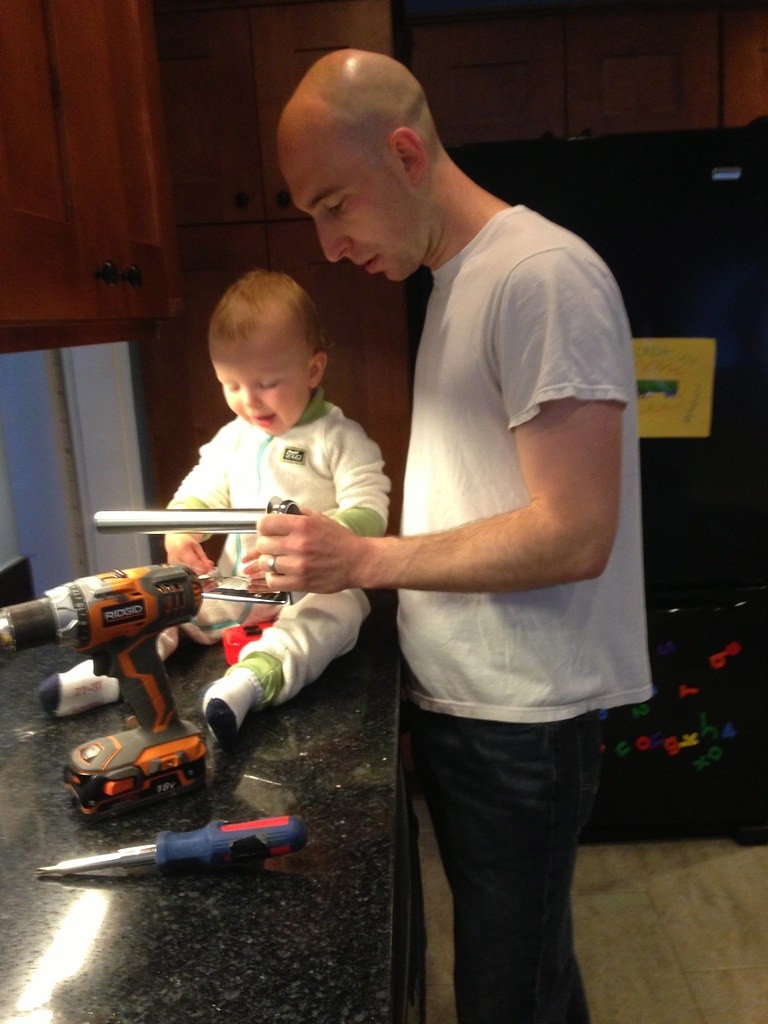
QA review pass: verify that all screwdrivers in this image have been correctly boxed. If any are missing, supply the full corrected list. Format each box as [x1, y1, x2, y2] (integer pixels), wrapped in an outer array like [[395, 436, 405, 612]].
[[37, 816, 310, 884]]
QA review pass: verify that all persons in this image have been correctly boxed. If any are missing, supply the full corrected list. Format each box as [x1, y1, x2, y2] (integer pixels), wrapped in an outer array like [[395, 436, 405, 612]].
[[241, 51, 653, 1024], [41, 273, 390, 738]]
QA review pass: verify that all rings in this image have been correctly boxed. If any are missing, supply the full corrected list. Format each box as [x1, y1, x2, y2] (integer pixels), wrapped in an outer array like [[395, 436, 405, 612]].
[[269, 556, 277, 574]]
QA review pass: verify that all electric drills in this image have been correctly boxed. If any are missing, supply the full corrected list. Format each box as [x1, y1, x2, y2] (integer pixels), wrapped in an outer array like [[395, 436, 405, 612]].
[[0, 561, 215, 820]]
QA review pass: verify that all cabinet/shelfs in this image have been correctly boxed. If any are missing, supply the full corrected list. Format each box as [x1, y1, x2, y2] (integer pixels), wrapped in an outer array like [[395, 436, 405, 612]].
[[1, 0, 767, 846]]
